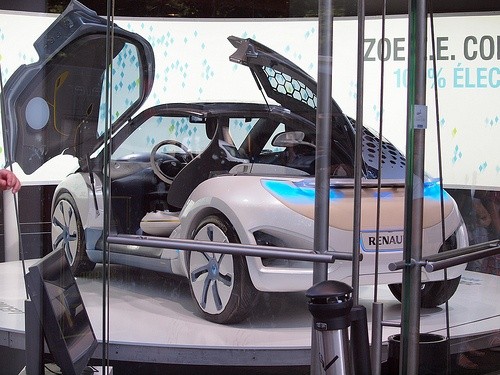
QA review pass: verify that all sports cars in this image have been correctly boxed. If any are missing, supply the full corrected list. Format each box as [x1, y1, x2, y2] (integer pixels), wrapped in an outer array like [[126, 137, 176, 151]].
[[0, 0, 471, 327]]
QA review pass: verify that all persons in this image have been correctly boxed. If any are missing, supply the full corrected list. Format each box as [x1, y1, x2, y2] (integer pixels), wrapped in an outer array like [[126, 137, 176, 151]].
[[0, 168, 22, 193]]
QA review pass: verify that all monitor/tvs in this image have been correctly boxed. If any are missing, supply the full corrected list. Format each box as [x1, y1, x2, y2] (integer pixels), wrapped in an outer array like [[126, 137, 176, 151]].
[[24, 246, 99, 375]]
